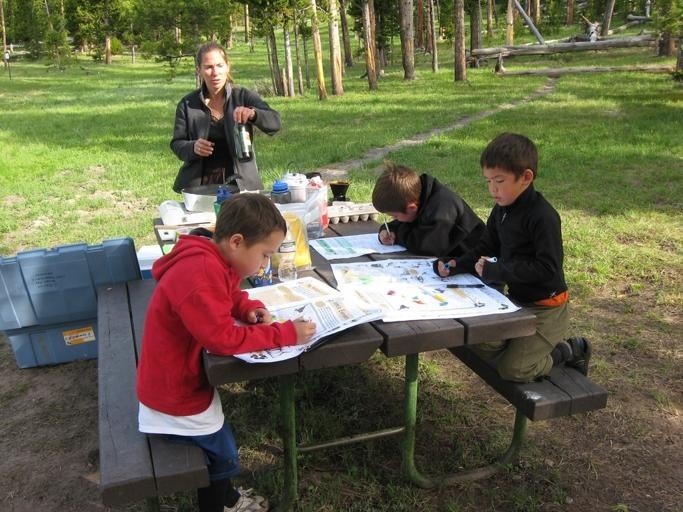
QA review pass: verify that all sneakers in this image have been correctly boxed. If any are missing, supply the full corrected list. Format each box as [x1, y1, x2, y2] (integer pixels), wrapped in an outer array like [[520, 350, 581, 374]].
[[555, 336, 591, 378], [222, 487, 269, 512]]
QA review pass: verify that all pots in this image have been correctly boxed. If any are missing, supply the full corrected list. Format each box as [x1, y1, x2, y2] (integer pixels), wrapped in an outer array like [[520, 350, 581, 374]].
[[181, 174, 243, 213]]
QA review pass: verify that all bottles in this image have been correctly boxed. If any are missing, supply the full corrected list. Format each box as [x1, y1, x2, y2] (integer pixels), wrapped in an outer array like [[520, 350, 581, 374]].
[[234, 120, 254, 162], [270, 179, 291, 203], [278, 223, 297, 282], [213, 187, 232, 217]]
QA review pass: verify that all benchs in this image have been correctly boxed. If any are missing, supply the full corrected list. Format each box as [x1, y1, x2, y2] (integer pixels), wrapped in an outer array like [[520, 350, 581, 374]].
[[297, 218, 606, 489], [97, 276, 213, 510]]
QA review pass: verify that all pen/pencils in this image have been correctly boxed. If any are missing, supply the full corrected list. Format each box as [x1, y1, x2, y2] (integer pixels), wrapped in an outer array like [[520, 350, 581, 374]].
[[447, 284, 485, 288], [487, 257, 497, 262], [303, 315, 310, 322], [384, 219, 394, 246], [418, 285, 444, 302]]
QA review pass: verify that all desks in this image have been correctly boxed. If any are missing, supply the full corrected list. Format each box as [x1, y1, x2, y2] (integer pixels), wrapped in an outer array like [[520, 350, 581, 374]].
[[151, 220, 384, 507]]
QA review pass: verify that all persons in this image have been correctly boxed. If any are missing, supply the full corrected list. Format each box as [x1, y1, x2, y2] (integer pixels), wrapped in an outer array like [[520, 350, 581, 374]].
[[371, 164, 486, 257], [432, 132, 592, 384], [168, 42, 281, 193], [135, 195, 315, 511]]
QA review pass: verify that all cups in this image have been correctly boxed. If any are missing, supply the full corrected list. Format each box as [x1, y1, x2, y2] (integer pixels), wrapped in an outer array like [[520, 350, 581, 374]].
[[305, 185, 329, 229], [329, 182, 349, 201]]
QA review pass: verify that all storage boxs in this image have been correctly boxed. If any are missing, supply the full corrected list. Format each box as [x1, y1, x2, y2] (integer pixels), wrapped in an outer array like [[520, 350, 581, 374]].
[[0, 238, 166, 370]]
